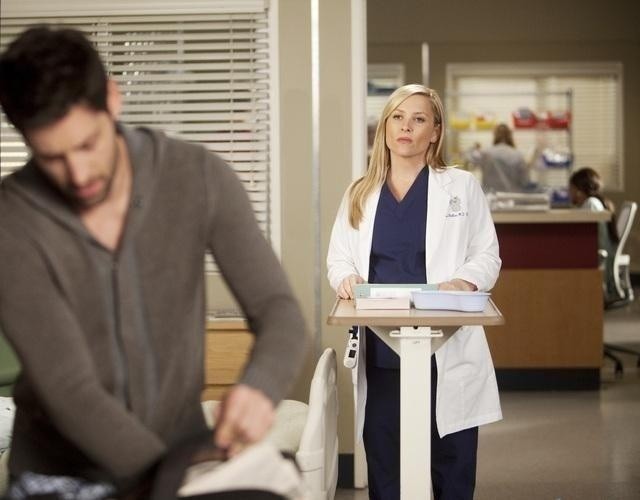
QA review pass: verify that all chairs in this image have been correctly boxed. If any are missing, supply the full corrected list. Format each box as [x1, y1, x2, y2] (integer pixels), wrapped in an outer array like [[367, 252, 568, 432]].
[[597, 199, 640, 378]]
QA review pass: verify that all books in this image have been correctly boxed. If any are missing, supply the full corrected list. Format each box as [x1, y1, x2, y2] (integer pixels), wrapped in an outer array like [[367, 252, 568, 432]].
[[492, 191, 552, 205], [491, 202, 550, 213]]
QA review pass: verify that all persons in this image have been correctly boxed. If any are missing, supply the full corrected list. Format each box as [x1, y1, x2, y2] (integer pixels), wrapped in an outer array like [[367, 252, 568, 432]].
[[0, 21, 313, 500], [463, 122, 531, 194], [326, 83, 503, 500], [569, 167, 622, 303]]
